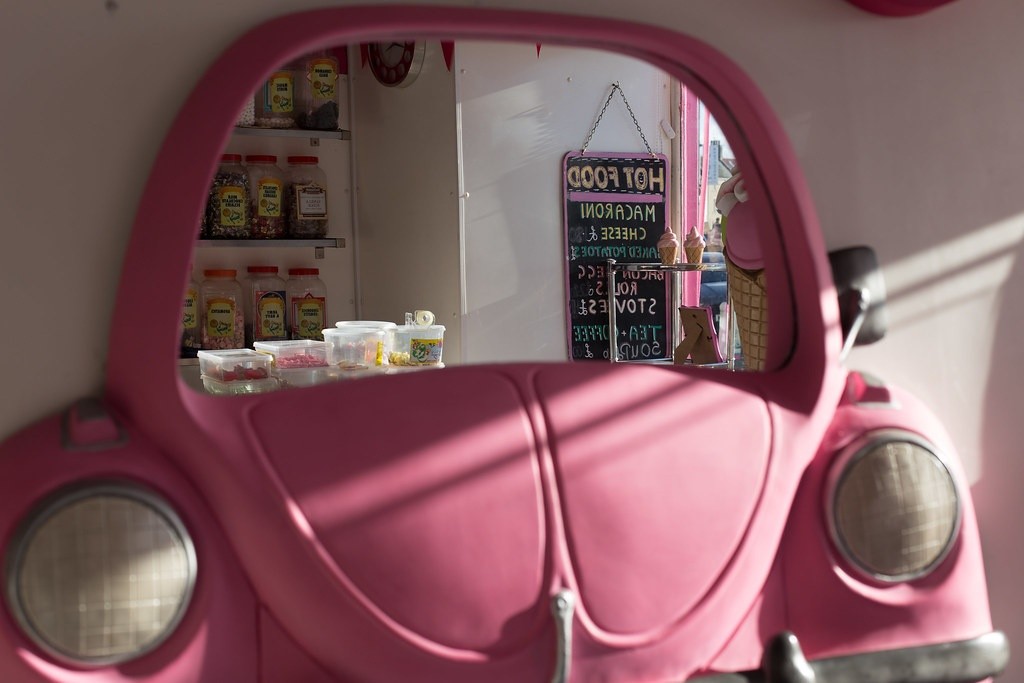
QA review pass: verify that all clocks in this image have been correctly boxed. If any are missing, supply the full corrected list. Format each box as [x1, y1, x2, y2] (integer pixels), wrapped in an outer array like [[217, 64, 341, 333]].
[[363, 40, 425, 90]]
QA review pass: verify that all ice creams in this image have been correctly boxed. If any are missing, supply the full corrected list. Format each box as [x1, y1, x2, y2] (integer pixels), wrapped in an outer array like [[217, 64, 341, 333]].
[[657, 226, 678, 265], [714, 163, 771, 369], [683, 226, 706, 264]]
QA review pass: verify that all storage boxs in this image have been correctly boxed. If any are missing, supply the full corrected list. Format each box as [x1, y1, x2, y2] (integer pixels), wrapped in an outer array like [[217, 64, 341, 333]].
[[196, 340, 334, 384]]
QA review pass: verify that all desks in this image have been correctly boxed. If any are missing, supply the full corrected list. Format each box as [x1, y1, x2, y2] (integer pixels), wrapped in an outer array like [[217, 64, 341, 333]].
[[178, 363, 445, 393]]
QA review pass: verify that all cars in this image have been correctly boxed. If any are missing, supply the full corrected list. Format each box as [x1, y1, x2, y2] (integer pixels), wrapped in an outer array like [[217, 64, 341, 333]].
[[699, 251, 730, 328]]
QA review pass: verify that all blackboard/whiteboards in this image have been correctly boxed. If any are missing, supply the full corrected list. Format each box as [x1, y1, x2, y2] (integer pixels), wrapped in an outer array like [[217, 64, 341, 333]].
[[562, 151, 673, 364]]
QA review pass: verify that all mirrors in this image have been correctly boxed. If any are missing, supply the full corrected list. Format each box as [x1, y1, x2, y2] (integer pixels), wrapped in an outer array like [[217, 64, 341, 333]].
[[173, 35, 797, 397]]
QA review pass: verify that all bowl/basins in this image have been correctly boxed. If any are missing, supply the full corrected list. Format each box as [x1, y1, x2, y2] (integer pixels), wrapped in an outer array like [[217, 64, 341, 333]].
[[335, 321, 397, 358], [253, 339, 334, 369], [394, 325, 445, 366], [321, 328, 385, 369], [196, 349, 273, 382]]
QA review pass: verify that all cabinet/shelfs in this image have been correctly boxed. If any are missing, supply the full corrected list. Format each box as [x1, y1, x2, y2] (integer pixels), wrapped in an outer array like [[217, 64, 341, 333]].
[[606, 259, 735, 372], [177, 127, 352, 366]]
